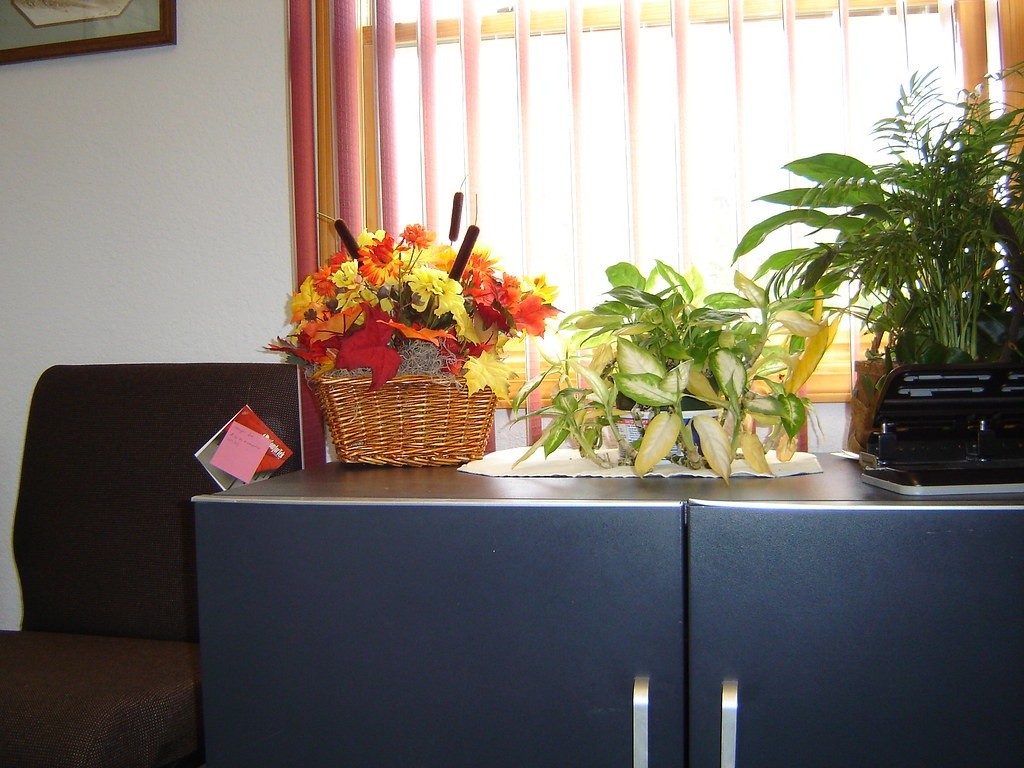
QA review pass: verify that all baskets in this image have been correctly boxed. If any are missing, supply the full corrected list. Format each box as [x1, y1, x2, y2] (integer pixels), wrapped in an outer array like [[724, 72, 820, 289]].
[[306, 365, 500, 467], [847, 279, 905, 453]]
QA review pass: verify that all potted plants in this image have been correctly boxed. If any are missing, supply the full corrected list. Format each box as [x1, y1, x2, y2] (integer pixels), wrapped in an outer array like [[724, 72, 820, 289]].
[[509, 258, 839, 486], [731, 61, 1024, 487]]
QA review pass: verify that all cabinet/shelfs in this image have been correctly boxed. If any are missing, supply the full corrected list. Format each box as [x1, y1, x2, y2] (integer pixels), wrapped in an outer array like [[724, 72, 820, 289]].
[[191, 403, 1024, 768]]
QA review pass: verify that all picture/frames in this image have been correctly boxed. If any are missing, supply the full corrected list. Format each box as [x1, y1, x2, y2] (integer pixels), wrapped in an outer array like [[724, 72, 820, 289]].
[[0, 0, 177, 65]]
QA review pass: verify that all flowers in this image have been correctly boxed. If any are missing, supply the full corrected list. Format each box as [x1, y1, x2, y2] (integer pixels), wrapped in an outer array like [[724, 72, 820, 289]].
[[266, 174, 565, 405]]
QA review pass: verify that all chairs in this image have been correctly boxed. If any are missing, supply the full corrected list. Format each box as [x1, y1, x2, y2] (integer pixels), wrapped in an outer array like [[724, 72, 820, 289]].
[[0, 363, 326, 768]]
